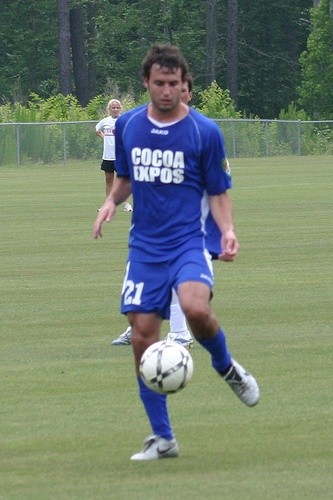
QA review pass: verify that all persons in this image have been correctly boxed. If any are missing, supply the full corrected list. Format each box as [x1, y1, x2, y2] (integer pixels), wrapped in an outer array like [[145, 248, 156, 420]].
[[96, 99, 133, 212], [93, 46, 259, 459], [112, 73, 194, 345]]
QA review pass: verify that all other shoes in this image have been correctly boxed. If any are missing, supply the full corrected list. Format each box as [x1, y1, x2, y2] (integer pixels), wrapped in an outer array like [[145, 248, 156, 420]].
[[123, 202, 132, 212]]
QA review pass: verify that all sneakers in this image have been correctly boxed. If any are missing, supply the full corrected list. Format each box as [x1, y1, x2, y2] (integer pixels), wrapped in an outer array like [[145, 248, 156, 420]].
[[221, 358, 259, 407], [111, 326, 130, 345], [129, 435, 177, 461], [163, 330, 193, 349]]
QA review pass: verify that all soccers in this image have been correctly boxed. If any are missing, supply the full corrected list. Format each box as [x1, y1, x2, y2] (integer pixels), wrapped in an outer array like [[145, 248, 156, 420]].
[[137, 339, 194, 395]]
